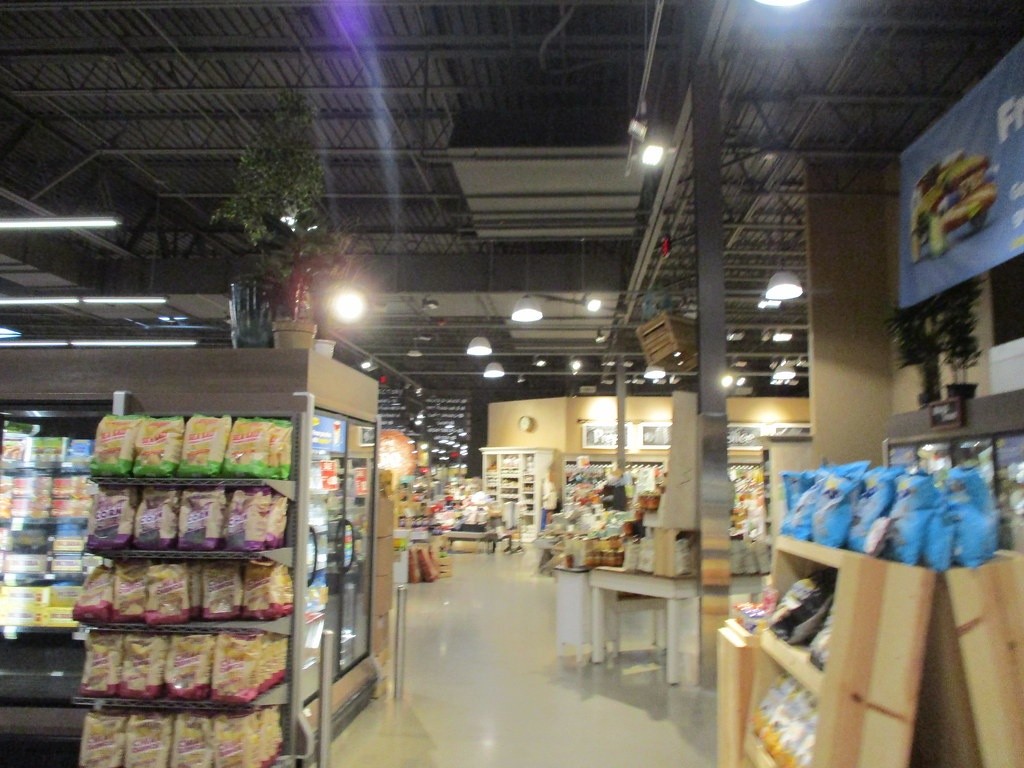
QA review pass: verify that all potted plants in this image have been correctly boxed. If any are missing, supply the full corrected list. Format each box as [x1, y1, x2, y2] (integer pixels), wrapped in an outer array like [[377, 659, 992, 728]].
[[309, 222, 348, 363], [216, 82, 332, 356], [894, 302, 942, 411], [934, 274, 983, 403]]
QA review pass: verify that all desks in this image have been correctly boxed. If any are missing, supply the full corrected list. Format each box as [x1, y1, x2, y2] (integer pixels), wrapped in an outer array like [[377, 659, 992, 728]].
[[585, 563, 702, 693]]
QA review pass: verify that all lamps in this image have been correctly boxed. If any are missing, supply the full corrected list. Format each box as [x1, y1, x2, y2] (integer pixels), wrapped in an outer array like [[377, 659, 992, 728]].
[[323, 107, 810, 396]]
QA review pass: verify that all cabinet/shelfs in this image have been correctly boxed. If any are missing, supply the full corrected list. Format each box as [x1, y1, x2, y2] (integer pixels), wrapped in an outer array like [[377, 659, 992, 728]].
[[74, 384, 318, 768], [881, 388, 1023, 507], [919, 547, 1024, 768], [713, 522, 934, 766], [293, 355, 380, 755], [553, 560, 671, 656], [0, 390, 134, 745], [380, 430, 771, 577]]
